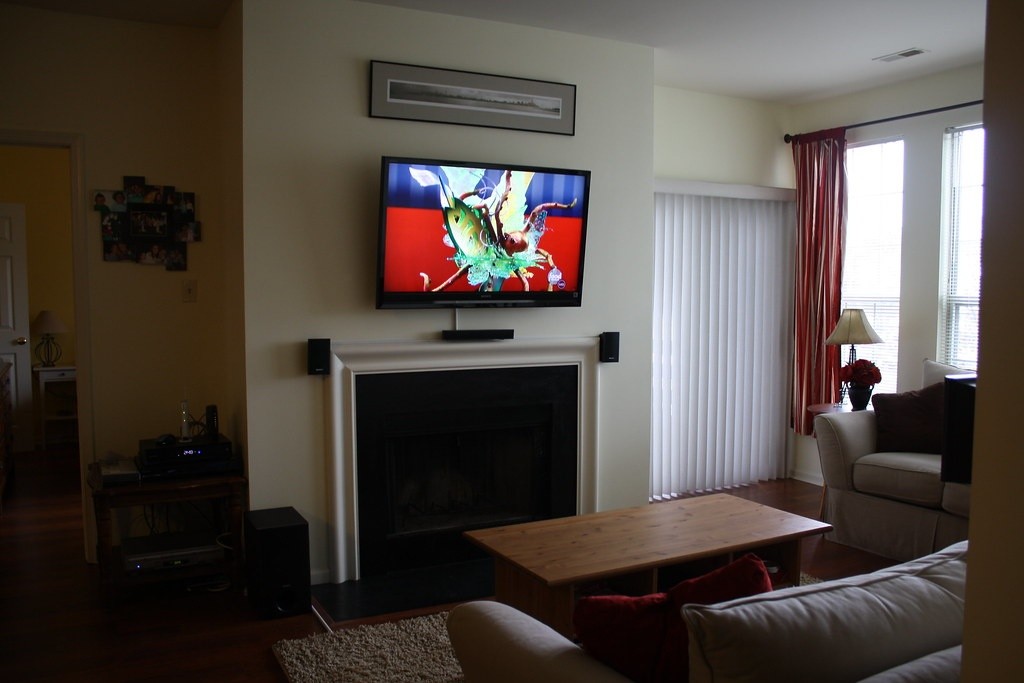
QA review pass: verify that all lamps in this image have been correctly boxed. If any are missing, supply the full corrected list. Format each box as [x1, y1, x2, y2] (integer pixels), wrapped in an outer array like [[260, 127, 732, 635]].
[[30, 311, 71, 367], [823, 309, 885, 407]]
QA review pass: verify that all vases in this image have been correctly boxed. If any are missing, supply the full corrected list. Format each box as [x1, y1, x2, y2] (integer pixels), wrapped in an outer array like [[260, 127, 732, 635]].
[[846, 381, 874, 411]]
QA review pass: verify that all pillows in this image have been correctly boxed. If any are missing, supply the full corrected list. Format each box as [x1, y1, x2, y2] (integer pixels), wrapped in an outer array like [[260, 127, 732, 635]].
[[572, 553, 772, 683], [872, 382, 945, 454], [682, 538, 972, 683]]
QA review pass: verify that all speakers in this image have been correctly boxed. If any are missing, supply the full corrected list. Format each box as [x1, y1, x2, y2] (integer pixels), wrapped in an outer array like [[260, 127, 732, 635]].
[[308, 338, 331, 375], [243, 506, 313, 620], [599, 332, 619, 362]]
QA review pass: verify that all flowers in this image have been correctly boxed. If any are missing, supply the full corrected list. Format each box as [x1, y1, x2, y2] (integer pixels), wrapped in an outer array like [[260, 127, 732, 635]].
[[839, 359, 881, 384]]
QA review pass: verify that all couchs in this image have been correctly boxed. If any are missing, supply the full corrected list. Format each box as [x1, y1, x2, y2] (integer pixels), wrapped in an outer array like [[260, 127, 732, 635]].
[[814, 358, 971, 562], [446, 600, 972, 683]]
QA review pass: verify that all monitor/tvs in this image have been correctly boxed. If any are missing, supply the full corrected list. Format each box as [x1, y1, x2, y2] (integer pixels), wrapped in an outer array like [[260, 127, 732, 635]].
[[375, 156, 592, 310]]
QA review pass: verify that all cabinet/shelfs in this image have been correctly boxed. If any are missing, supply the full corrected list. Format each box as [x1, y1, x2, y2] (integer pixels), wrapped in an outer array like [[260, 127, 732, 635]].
[[88, 462, 244, 609]]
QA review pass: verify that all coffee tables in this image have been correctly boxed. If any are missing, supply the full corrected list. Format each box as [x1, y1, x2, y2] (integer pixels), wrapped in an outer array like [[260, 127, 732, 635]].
[[462, 493, 834, 641]]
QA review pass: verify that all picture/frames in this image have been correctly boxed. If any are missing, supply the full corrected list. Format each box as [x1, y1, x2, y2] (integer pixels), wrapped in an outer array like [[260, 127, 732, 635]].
[[368, 59, 577, 136]]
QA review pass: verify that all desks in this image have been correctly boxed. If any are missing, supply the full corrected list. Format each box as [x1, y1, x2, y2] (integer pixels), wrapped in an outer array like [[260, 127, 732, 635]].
[[33, 363, 78, 449]]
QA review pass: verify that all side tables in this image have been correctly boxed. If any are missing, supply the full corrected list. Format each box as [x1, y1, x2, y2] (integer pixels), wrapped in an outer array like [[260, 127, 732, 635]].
[[808, 403, 874, 536]]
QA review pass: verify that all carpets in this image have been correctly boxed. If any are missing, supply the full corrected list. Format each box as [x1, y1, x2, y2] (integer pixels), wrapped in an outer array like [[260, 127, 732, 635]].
[[271, 570, 824, 683]]
